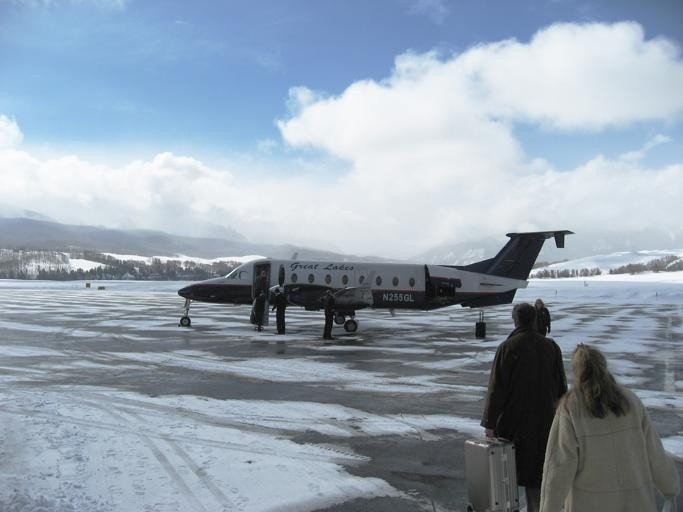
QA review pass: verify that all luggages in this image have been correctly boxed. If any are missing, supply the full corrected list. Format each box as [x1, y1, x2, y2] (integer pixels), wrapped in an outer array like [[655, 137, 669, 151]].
[[464, 437, 519, 512], [476, 310, 486, 338]]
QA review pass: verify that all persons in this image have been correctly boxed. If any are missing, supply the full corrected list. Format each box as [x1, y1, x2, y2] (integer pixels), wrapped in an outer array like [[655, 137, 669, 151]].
[[540, 343, 683, 512], [254, 292, 268, 334], [319, 289, 337, 340], [535, 299, 550, 337], [253, 271, 268, 297], [480, 302, 568, 512], [272, 289, 287, 334]]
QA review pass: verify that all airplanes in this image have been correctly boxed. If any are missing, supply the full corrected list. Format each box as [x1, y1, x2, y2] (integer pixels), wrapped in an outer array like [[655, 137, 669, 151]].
[[178, 229, 575, 333]]
